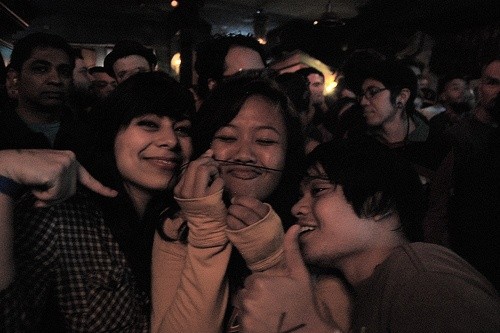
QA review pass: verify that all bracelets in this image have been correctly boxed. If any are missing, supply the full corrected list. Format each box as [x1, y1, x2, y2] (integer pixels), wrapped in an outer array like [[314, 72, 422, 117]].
[[0, 175, 25, 201]]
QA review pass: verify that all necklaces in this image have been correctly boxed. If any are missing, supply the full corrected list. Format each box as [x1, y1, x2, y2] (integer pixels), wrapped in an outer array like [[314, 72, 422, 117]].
[[377, 112, 409, 150]]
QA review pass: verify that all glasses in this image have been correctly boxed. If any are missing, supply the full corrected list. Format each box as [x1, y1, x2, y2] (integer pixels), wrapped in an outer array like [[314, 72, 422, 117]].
[[478, 72, 500, 86], [355, 85, 386, 99]]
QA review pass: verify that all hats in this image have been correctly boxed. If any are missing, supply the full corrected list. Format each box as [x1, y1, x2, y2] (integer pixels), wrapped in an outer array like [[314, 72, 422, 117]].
[[103, 39, 157, 78]]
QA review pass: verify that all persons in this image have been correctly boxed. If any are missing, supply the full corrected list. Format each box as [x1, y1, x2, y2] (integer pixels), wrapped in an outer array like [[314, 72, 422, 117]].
[[6, 47, 482, 244], [292, 136, 500, 333], [425, 60, 500, 291], [0, 54, 118, 291], [16, 72, 196, 333], [152, 76, 350, 333]]
